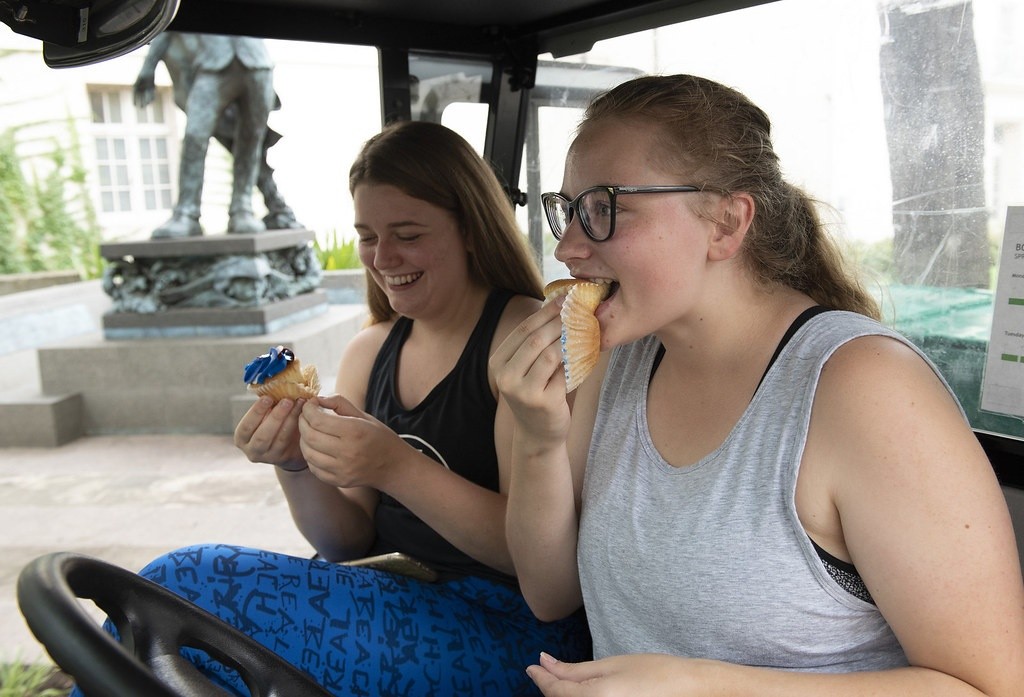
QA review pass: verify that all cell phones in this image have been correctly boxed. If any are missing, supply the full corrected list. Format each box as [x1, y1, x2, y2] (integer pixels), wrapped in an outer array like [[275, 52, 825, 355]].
[[338, 552, 437, 583]]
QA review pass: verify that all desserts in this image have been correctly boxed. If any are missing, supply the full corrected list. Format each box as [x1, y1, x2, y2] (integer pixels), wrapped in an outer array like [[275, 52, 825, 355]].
[[543, 277, 611, 315], [243, 344, 309, 400]]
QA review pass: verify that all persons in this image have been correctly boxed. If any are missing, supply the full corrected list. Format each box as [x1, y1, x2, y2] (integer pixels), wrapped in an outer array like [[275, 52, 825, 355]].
[[490, 74, 1024, 697], [72, 121, 575, 697], [149, 33, 276, 240]]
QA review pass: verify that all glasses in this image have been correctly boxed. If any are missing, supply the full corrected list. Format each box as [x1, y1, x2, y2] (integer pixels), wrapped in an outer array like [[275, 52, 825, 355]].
[[541, 186, 739, 244]]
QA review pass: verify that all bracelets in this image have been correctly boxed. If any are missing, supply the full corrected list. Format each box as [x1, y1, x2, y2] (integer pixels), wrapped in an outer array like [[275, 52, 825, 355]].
[[281, 465, 309, 472]]
